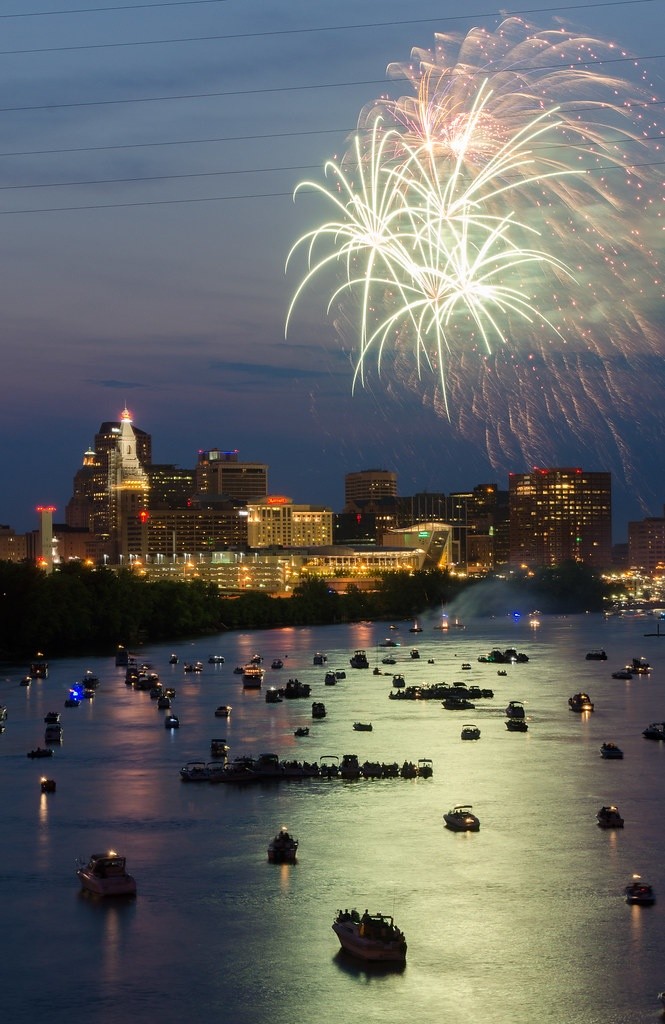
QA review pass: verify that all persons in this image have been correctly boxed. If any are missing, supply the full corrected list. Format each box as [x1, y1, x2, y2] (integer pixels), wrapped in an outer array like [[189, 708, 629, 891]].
[[361, 909, 371, 931], [338, 909, 350, 922], [279, 831, 289, 840]]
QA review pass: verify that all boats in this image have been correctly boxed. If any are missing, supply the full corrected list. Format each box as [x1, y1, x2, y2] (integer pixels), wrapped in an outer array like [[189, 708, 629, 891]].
[[612, 671, 632, 679], [596, 806, 625, 828], [331, 915, 407, 961], [266, 834, 299, 860], [18, 609, 529, 793], [625, 882, 656, 903], [642, 722, 665, 740], [625, 658, 651, 674], [76, 854, 137, 895], [443, 805, 481, 829], [527, 607, 665, 620], [586, 652, 607, 660], [568, 693, 594, 711], [600, 742, 625, 760]]
